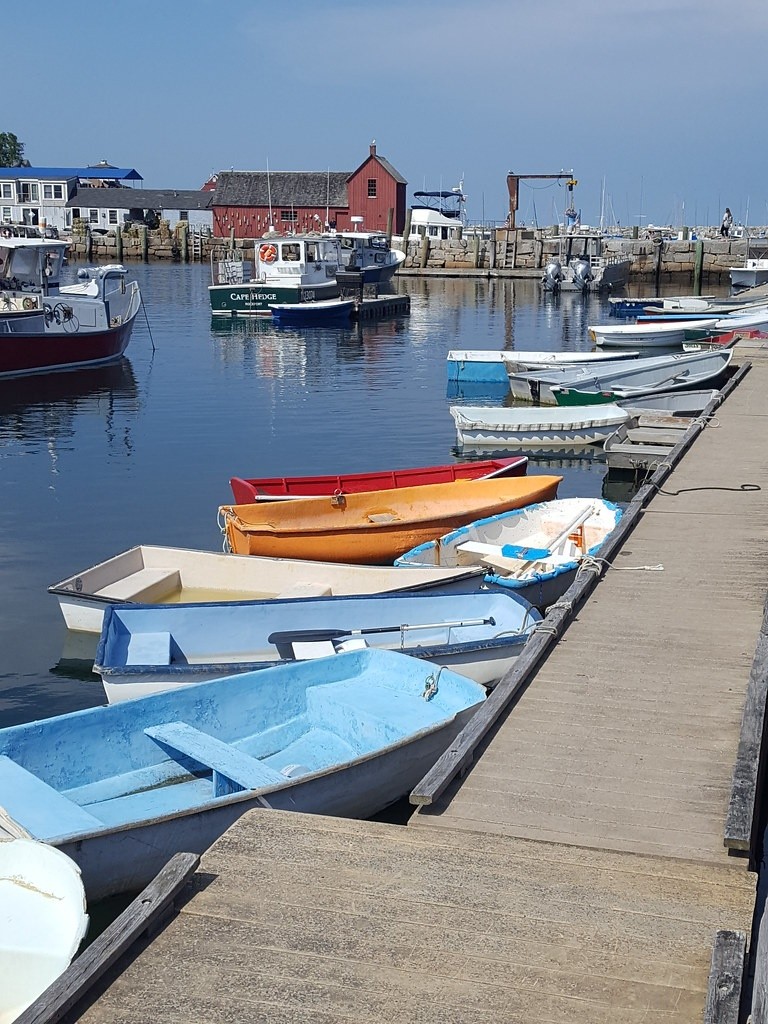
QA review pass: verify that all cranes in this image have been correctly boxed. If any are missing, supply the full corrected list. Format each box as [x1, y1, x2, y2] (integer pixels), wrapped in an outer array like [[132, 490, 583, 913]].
[[506, 170, 578, 229]]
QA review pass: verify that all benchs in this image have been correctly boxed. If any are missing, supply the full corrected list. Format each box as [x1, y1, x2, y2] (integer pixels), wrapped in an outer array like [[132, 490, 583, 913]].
[[611, 384, 651, 391], [111, 631, 172, 667], [610, 443, 675, 454], [674, 375, 701, 380], [289, 638, 337, 661], [1, 754, 106, 840], [272, 583, 333, 599], [455, 540, 580, 568], [93, 568, 181, 604], [144, 721, 290, 799], [626, 428, 687, 444], [367, 511, 400, 524], [306, 681, 447, 756]]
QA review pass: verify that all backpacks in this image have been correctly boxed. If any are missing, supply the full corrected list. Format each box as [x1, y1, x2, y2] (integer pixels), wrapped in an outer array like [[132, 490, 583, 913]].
[[727, 214, 733, 224]]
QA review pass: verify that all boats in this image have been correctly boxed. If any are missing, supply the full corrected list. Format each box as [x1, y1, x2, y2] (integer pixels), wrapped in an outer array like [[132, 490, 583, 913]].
[[229, 454, 529, 507], [550, 347, 735, 405], [728, 258, 768, 289], [602, 415, 698, 471], [47, 544, 499, 636], [1, 836, 91, 1024], [0, 645, 487, 901], [208, 157, 408, 320], [0, 225, 141, 379], [587, 280, 768, 353], [446, 350, 641, 382], [219, 474, 565, 557], [395, 496, 625, 609], [613, 388, 720, 417], [90, 587, 545, 707], [448, 403, 629, 462], [508, 350, 710, 404]]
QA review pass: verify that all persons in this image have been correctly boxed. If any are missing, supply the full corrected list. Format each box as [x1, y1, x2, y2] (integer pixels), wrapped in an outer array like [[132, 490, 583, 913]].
[[720, 208, 732, 239], [329, 217, 337, 233]]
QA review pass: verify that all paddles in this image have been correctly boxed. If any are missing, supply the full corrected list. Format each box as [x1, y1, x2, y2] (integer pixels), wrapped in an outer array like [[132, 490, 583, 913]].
[[266, 615, 496, 645]]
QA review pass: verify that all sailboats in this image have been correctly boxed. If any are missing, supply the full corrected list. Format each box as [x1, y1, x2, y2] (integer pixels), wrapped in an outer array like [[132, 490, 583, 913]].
[[403, 173, 768, 240]]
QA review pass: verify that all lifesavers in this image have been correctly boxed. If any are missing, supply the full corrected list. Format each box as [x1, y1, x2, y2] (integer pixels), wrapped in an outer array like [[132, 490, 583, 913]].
[[259, 244, 278, 263]]
